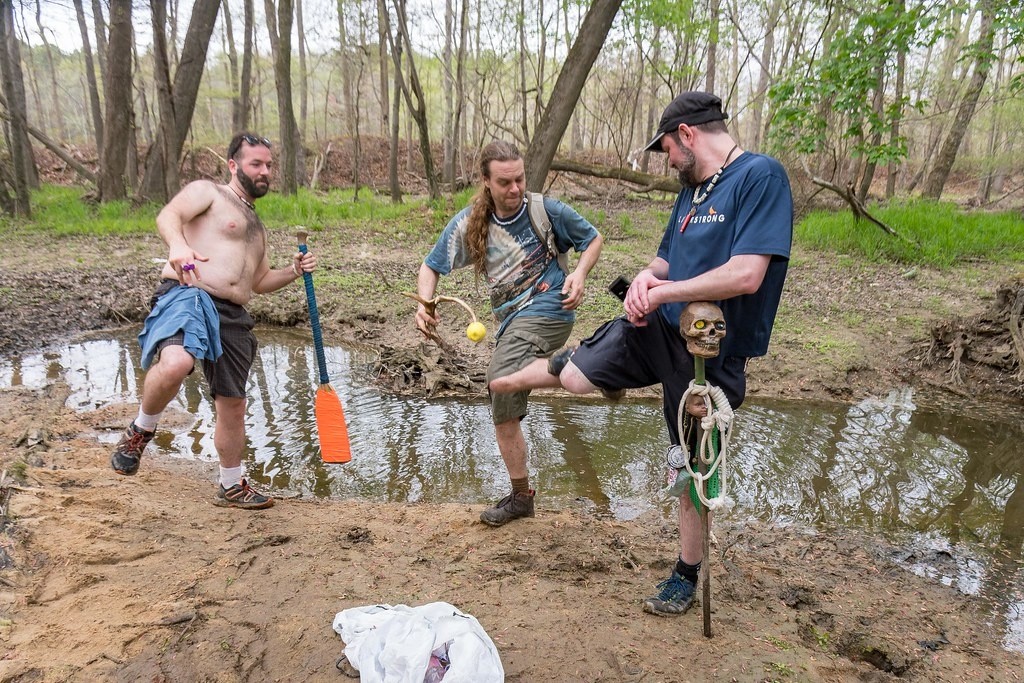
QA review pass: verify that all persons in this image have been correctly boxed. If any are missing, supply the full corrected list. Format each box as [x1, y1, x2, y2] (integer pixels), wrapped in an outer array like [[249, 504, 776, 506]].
[[416, 141, 602, 526], [111, 132, 316, 509], [548, 91, 794, 619]]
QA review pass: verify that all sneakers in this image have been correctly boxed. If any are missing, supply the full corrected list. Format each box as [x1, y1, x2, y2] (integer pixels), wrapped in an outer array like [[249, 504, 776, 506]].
[[642, 570, 698, 618], [110, 418, 157, 476], [212, 479, 274, 509], [479, 488, 538, 527], [594, 381, 629, 405], [548, 337, 584, 377]]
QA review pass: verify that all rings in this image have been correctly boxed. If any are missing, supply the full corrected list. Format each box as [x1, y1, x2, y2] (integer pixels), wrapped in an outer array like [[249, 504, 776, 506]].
[[181, 263, 195, 271]]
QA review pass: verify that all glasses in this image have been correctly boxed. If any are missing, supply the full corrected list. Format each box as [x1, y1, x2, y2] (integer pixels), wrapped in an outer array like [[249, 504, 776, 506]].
[[231, 135, 272, 160]]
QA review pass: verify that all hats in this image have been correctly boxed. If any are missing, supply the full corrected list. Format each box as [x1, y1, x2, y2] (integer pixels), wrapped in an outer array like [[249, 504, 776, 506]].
[[642, 92, 728, 153]]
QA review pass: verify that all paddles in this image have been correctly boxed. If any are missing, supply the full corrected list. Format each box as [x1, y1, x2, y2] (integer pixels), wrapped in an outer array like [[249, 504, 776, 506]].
[[287, 224, 354, 465]]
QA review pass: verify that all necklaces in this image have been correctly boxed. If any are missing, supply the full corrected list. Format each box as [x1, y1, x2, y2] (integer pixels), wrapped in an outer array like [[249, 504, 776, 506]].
[[227, 184, 256, 209], [680, 145, 738, 232], [492, 195, 528, 226]]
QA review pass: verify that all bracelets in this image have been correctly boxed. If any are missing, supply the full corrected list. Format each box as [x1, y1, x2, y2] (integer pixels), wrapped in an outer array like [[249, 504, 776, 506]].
[[293, 263, 302, 277]]
